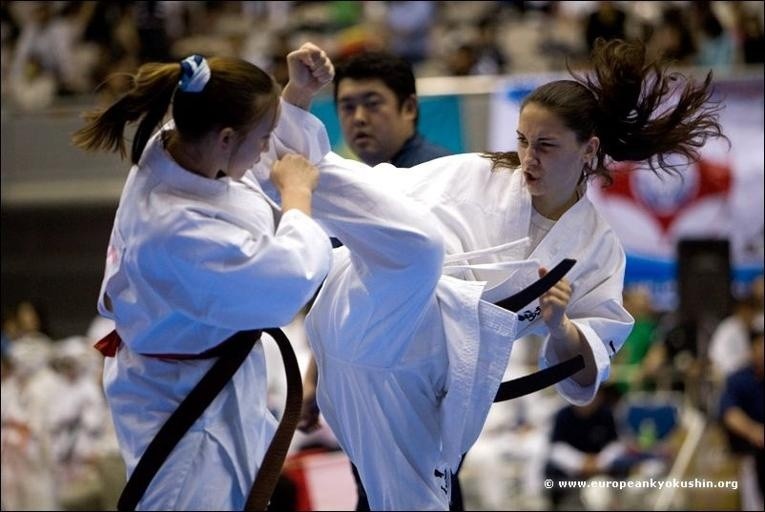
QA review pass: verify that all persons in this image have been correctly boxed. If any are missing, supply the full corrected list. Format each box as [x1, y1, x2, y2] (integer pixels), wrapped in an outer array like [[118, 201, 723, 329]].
[[253, 37, 733, 512], [74, 37, 336, 510], [291, 48, 466, 509]]
